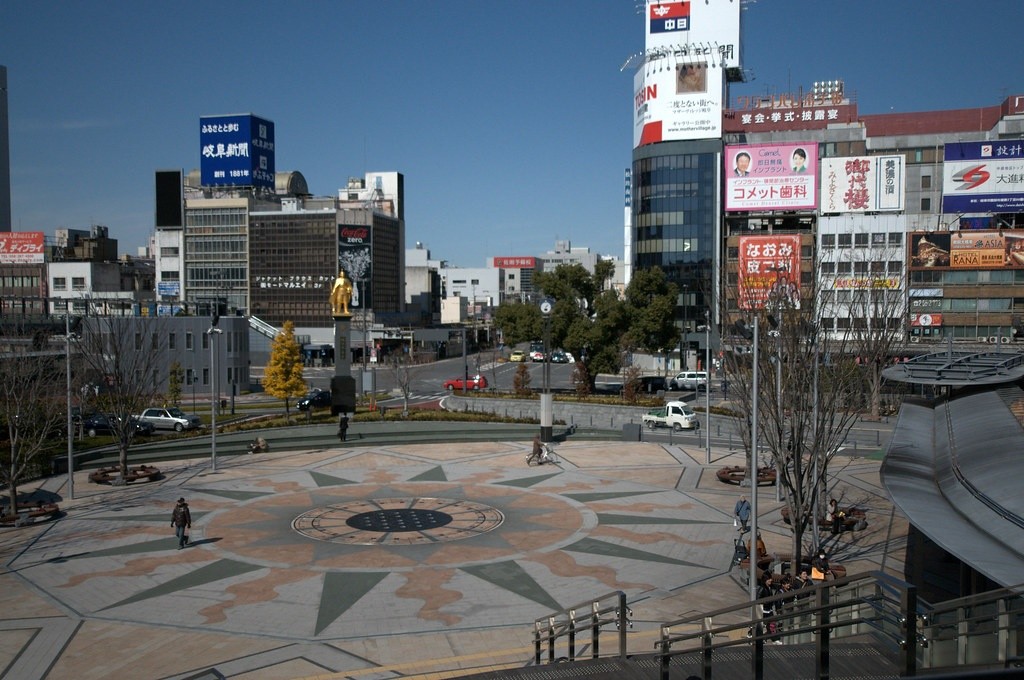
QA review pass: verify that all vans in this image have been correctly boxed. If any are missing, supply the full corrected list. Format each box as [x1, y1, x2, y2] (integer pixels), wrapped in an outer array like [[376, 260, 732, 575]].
[[669, 370, 711, 391]]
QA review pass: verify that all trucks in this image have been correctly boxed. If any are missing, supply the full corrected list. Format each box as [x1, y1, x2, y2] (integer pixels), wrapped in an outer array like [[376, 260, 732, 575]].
[[641, 400, 700, 432]]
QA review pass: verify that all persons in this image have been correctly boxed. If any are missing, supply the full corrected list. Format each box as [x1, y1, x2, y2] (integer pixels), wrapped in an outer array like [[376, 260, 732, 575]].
[[814, 549, 829, 572], [747, 570, 815, 645], [827, 499, 842, 533], [526, 434, 545, 465], [787, 436, 794, 464], [171, 498, 191, 550], [331, 271, 352, 314], [881, 397, 901, 414], [735, 494, 751, 533], [339, 413, 349, 441], [734, 153, 750, 176], [769, 276, 801, 309], [792, 149, 806, 173], [247, 438, 267, 454], [747, 532, 766, 557]]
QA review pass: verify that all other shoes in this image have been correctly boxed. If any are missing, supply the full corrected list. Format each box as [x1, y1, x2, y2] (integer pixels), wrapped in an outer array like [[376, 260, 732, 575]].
[[185, 536, 189, 544], [746, 634, 753, 638], [177, 546, 180, 550], [771, 640, 782, 646]]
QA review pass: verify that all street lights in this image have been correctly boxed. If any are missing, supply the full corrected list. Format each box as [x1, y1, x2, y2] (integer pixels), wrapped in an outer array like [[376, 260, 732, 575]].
[[64, 332, 77, 500], [731, 315, 761, 642], [697, 310, 712, 464], [207, 328, 224, 470]]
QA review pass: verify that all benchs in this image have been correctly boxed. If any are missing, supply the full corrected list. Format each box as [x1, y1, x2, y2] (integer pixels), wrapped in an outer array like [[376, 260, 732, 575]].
[[716, 468, 777, 486], [781, 505, 866, 531], [0, 493, 63, 520], [88, 467, 160, 485], [739, 554, 846, 588]]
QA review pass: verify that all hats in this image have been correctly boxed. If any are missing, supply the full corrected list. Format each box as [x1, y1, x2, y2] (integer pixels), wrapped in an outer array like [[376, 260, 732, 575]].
[[816, 549, 826, 556], [178, 497, 185, 503]]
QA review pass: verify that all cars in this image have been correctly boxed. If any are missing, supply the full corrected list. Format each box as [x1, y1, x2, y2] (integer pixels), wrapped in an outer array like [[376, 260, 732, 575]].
[[632, 375, 671, 393], [139, 406, 200, 432], [296, 390, 333, 411], [530, 343, 573, 363], [81, 410, 156, 436], [443, 374, 488, 390], [510, 350, 526, 362]]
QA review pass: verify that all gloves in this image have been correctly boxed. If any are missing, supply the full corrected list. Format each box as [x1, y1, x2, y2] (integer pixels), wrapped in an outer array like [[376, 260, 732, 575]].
[[187, 524, 191, 528], [171, 523, 174, 528]]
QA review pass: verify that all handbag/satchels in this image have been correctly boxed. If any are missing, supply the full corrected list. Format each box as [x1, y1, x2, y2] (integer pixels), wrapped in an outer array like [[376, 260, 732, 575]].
[[336, 432, 341, 437]]
